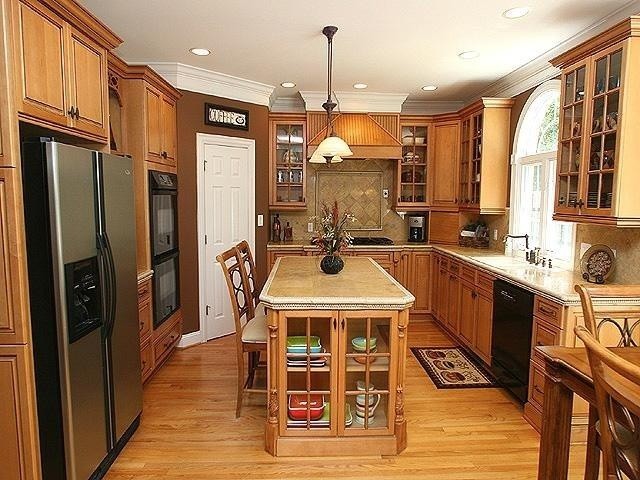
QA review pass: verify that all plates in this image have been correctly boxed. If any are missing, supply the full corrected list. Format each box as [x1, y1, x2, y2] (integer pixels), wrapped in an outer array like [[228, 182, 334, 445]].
[[569, 191, 613, 209], [286, 349, 330, 368], [285, 401, 352, 428], [580, 243, 617, 282]]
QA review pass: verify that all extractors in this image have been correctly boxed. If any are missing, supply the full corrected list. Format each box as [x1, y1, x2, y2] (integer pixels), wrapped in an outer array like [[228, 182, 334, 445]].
[[306, 112, 403, 162]]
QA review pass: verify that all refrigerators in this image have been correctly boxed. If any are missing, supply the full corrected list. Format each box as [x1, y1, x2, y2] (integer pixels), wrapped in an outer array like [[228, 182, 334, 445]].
[[20, 135, 144, 480]]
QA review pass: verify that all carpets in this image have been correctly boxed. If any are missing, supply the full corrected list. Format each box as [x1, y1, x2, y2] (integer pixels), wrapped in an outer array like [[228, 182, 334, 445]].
[[409, 345, 504, 389]]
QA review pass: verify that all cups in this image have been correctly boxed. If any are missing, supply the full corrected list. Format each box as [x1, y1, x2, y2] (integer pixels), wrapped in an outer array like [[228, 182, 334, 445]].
[[570, 112, 618, 138], [401, 196, 421, 201], [575, 150, 615, 170]]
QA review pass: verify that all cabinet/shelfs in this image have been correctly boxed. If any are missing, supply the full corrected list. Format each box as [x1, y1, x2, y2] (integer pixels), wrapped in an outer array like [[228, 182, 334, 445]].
[[138, 268, 157, 386], [268, 245, 495, 369], [521, 291, 640, 449], [430, 111, 462, 215], [1, 1, 124, 146], [267, 110, 309, 212], [260, 302, 412, 458], [153, 306, 185, 373], [391, 114, 432, 213], [458, 95, 516, 217], [126, 62, 184, 175]]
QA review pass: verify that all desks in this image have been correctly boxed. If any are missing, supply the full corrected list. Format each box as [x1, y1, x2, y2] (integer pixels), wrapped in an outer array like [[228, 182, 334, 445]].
[[531, 340, 640, 480]]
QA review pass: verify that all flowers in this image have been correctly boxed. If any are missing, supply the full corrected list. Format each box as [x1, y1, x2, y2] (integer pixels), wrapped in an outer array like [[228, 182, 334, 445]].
[[302, 196, 362, 254]]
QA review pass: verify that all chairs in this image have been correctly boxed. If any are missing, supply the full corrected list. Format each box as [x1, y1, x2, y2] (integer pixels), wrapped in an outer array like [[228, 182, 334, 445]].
[[570, 320, 640, 480], [232, 240, 269, 386], [214, 245, 267, 420], [571, 279, 640, 480]]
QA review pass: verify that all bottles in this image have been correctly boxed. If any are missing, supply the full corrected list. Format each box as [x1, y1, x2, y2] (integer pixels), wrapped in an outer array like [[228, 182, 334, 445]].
[[273, 217, 280, 243], [537, 250, 541, 264], [277, 170, 302, 183], [284, 222, 293, 242]]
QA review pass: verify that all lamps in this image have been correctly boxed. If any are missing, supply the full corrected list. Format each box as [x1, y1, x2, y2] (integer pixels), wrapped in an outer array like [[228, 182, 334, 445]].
[[306, 24, 353, 167]]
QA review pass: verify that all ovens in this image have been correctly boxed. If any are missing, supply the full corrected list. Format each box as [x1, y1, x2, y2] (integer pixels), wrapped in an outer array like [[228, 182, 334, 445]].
[[148, 168, 181, 332]]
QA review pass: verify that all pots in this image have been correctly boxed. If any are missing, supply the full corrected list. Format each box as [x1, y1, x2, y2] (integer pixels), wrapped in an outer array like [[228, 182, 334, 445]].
[[402, 170, 421, 183]]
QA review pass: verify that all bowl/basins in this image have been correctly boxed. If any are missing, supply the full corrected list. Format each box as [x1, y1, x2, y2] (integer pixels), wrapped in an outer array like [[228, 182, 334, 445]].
[[287, 392, 327, 418], [351, 336, 377, 364], [287, 335, 323, 361]]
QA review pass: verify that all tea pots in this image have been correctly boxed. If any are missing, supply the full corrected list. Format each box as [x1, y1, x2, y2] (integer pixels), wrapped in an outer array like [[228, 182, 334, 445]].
[[282, 147, 299, 167], [356, 380, 381, 427]]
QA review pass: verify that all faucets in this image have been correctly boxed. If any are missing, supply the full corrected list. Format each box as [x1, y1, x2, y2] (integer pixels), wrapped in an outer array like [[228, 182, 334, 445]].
[[502, 233, 529, 261]]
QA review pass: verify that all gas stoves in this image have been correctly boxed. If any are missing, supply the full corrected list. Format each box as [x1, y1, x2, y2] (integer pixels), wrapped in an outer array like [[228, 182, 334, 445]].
[[310, 236, 394, 247]]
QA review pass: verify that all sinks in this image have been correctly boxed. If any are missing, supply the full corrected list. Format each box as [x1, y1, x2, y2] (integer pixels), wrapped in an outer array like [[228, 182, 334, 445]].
[[467, 254, 525, 269]]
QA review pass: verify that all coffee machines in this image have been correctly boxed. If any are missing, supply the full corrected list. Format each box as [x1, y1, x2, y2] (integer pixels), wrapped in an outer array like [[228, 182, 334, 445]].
[[408, 215, 425, 243]]
